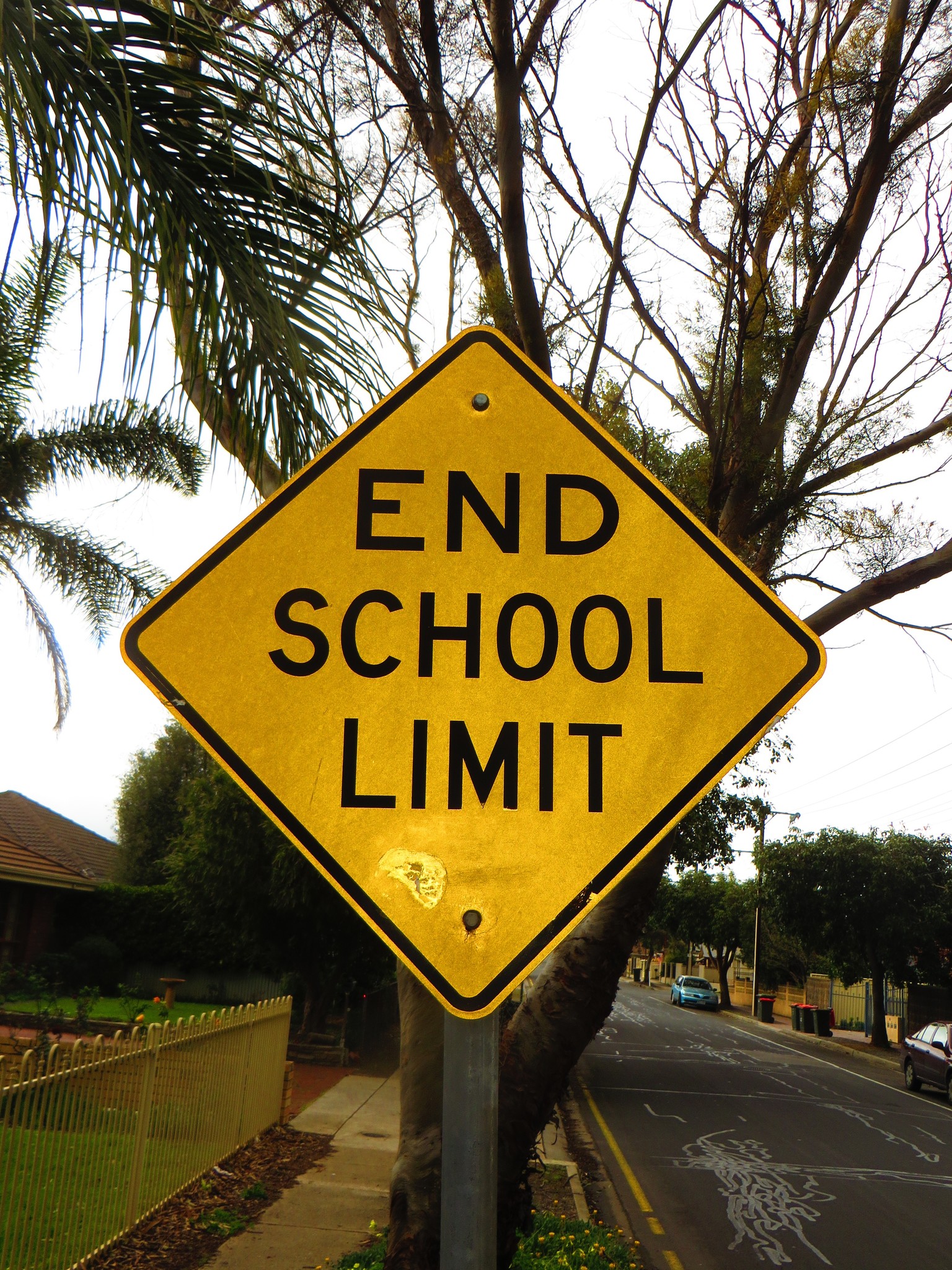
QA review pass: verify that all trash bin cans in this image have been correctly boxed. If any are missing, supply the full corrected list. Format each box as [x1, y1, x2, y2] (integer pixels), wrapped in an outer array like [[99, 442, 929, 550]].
[[633, 968, 643, 982], [756, 993, 777, 1017], [789, 1003, 806, 1031], [758, 998, 776, 1023], [797, 1004, 819, 1033], [810, 1008, 833, 1037]]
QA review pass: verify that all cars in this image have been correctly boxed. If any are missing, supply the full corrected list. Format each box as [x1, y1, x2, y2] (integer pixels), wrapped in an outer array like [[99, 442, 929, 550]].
[[670, 975, 719, 1012], [897, 1020, 951, 1107]]
